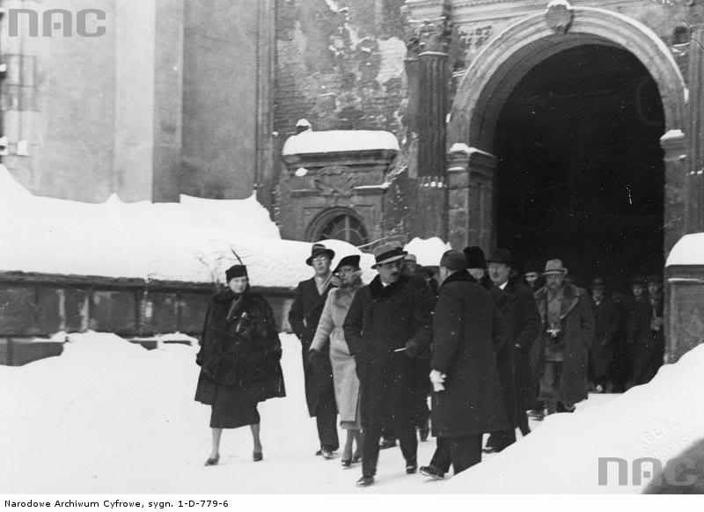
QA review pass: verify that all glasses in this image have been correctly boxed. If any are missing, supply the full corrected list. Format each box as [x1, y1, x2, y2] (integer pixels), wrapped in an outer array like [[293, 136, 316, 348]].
[[312, 257, 328, 261]]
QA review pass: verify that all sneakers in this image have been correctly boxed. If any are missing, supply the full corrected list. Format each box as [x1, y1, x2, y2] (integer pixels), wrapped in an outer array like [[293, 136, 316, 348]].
[[420, 466, 444, 480]]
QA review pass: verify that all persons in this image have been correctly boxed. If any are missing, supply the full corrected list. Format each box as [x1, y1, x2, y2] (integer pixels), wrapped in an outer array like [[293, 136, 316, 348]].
[[288, 240, 665, 484], [195, 265, 285, 467]]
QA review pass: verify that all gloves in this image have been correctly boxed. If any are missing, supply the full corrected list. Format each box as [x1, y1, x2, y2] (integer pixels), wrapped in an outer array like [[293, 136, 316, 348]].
[[429, 369, 446, 392], [309, 350, 320, 366]]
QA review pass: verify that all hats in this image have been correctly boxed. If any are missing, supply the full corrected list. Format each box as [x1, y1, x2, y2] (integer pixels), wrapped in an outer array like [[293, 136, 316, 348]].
[[522, 263, 539, 273], [226, 265, 248, 283], [403, 254, 422, 267], [589, 277, 607, 289], [488, 248, 513, 265], [541, 260, 568, 275], [371, 244, 405, 269], [439, 250, 464, 270], [333, 255, 359, 274], [306, 244, 334, 265], [463, 247, 486, 269]]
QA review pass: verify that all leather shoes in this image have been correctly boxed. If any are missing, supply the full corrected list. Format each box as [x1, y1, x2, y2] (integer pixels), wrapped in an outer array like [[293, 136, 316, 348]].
[[355, 477, 374, 486], [317, 442, 338, 456], [420, 419, 429, 440], [321, 445, 333, 458], [379, 439, 395, 449], [207, 454, 218, 465], [406, 459, 417, 472], [352, 453, 362, 463], [342, 457, 351, 467], [253, 446, 261, 461]]
